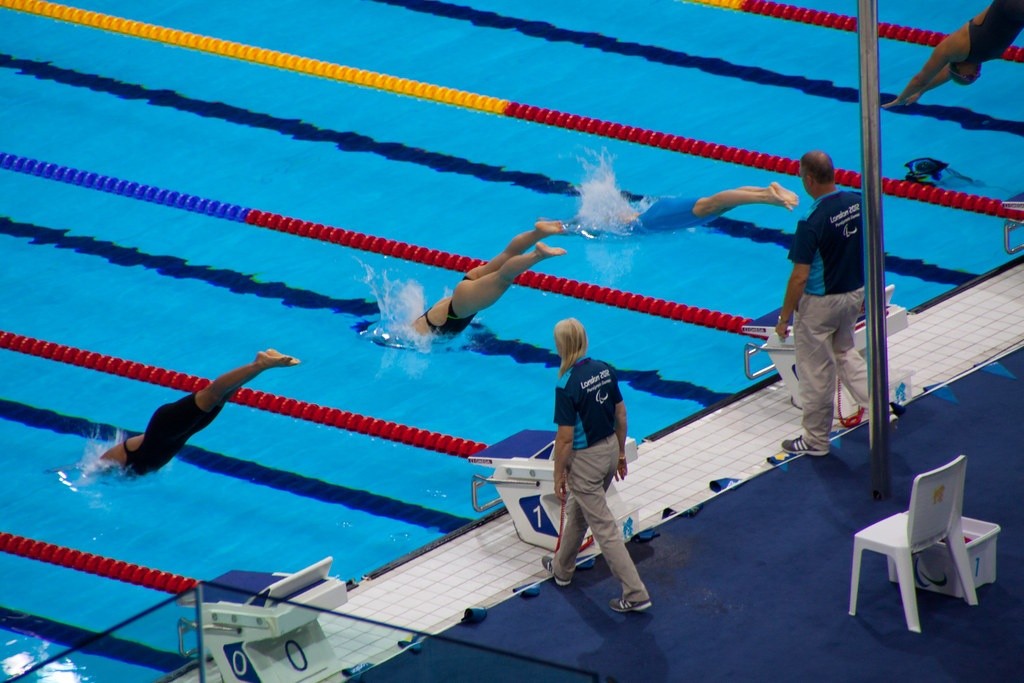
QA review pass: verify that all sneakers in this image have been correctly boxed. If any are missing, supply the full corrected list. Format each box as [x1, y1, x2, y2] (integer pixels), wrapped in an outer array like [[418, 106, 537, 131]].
[[781, 435, 830, 457], [542, 555, 571, 587], [889, 410, 899, 434], [608, 598, 653, 612]]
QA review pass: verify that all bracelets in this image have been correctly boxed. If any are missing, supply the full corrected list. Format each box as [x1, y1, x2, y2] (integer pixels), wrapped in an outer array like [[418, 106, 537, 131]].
[[777, 316, 789, 324], [619, 456, 626, 461]]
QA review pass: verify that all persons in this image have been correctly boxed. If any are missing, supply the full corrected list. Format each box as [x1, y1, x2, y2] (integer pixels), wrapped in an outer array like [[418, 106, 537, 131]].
[[96, 348, 300, 477], [542, 318, 653, 612], [624, 182, 798, 234], [775, 151, 899, 457], [409, 221, 567, 341], [881, 0, 1024, 109]]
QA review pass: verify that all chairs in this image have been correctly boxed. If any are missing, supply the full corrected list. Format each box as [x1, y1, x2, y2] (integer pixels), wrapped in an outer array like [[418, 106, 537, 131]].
[[848, 455, 978, 636]]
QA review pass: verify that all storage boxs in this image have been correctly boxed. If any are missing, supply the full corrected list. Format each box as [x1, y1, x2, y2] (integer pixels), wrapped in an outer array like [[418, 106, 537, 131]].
[[917, 516, 1000, 598]]
